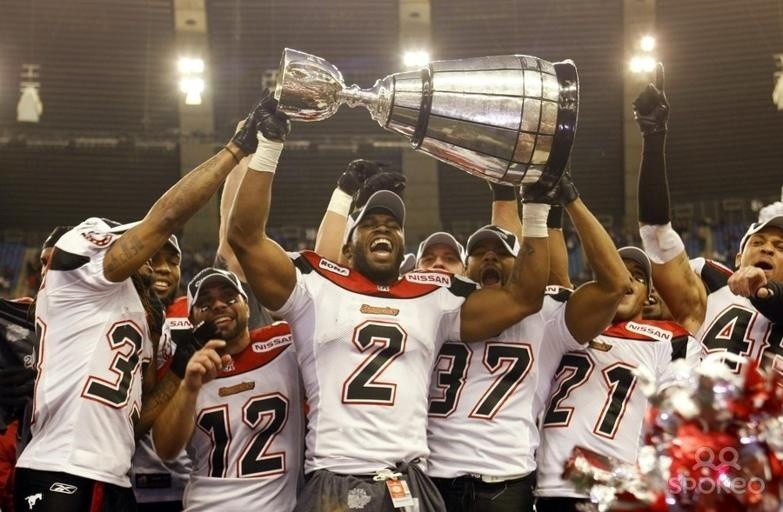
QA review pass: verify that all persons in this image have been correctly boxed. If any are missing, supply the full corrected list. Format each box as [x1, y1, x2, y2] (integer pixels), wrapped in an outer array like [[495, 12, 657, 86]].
[[3, 94, 270, 511], [631, 60, 781, 511], [224, 108, 556, 510]]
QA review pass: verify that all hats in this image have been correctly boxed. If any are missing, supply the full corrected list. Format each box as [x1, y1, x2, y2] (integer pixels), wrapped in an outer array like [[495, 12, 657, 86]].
[[341, 189, 405, 243], [169, 235, 181, 254], [545, 285, 573, 297], [616, 246, 652, 299], [740, 214, 783, 253], [99, 217, 144, 232], [416, 231, 464, 265], [185, 267, 249, 326], [465, 224, 521, 256]]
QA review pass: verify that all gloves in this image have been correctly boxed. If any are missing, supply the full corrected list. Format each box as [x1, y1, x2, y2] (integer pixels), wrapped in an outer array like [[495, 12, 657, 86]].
[[632, 62, 671, 135], [554, 157, 580, 205], [232, 88, 278, 151], [518, 182, 553, 204], [261, 110, 292, 142], [488, 180, 516, 202], [355, 171, 407, 205], [338, 158, 391, 197]]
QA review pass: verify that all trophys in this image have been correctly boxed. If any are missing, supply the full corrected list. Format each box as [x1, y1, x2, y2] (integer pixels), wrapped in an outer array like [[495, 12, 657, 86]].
[[271, 47, 579, 198]]
